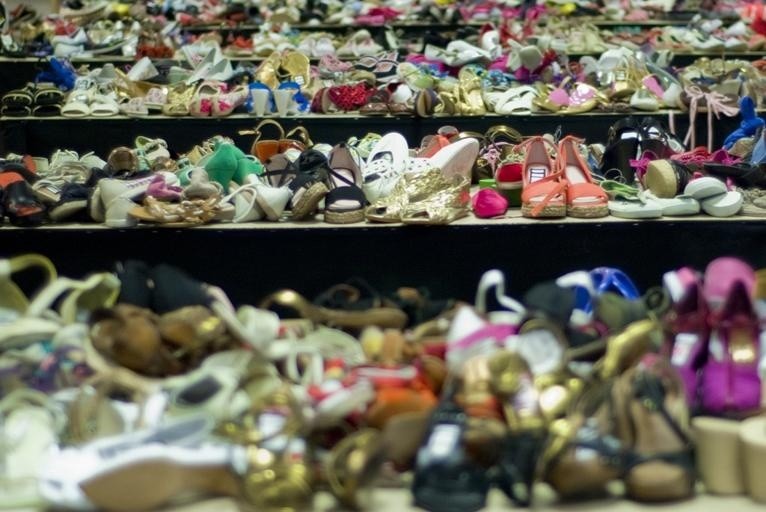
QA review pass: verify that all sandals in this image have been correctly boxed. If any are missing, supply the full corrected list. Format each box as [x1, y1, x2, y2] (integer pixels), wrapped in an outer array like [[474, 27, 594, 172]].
[[0, 0, 761, 221], [0, 249, 761, 512]]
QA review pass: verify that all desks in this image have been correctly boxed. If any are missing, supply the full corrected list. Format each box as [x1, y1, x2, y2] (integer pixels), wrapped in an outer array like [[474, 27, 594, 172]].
[[0, 0, 766, 507]]
